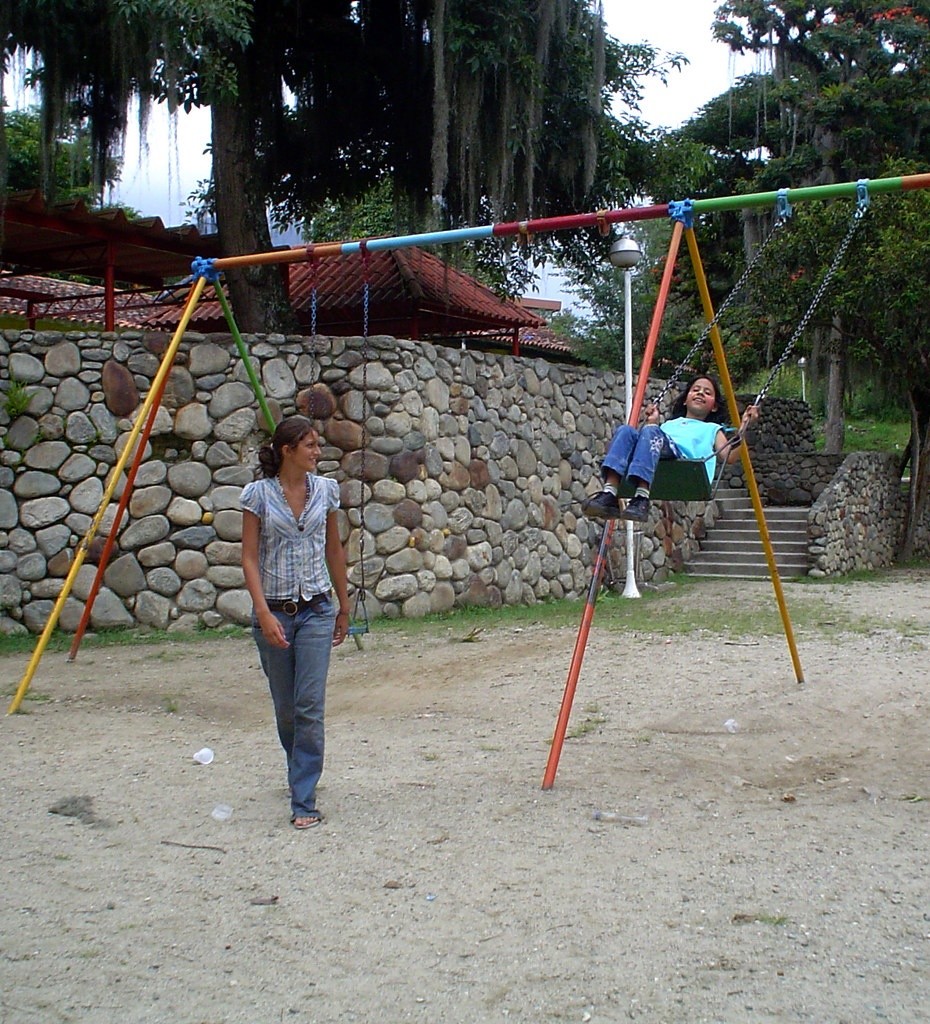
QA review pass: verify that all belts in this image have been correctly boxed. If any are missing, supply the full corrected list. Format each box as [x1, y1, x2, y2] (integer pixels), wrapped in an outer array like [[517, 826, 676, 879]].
[[266, 589, 334, 617]]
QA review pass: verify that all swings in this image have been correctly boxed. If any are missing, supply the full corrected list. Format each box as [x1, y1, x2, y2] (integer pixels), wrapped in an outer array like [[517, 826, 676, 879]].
[[593, 200, 873, 504], [305, 255, 373, 636]]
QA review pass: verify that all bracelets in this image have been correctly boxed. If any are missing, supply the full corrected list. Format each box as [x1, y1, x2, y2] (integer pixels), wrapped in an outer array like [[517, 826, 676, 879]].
[[340, 612, 349, 615]]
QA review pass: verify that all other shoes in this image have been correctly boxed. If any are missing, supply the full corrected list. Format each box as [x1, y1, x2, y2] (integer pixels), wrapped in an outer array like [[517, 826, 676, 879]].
[[286, 786, 293, 798], [293, 817, 321, 828]]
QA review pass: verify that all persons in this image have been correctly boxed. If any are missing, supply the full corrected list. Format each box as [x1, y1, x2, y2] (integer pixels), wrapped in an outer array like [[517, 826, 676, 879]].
[[236, 417, 351, 830], [582, 375, 760, 523]]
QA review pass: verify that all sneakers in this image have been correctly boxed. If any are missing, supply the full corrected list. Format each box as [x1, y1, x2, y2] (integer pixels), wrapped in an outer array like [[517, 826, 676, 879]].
[[581, 491, 621, 518], [621, 494, 649, 522]]
